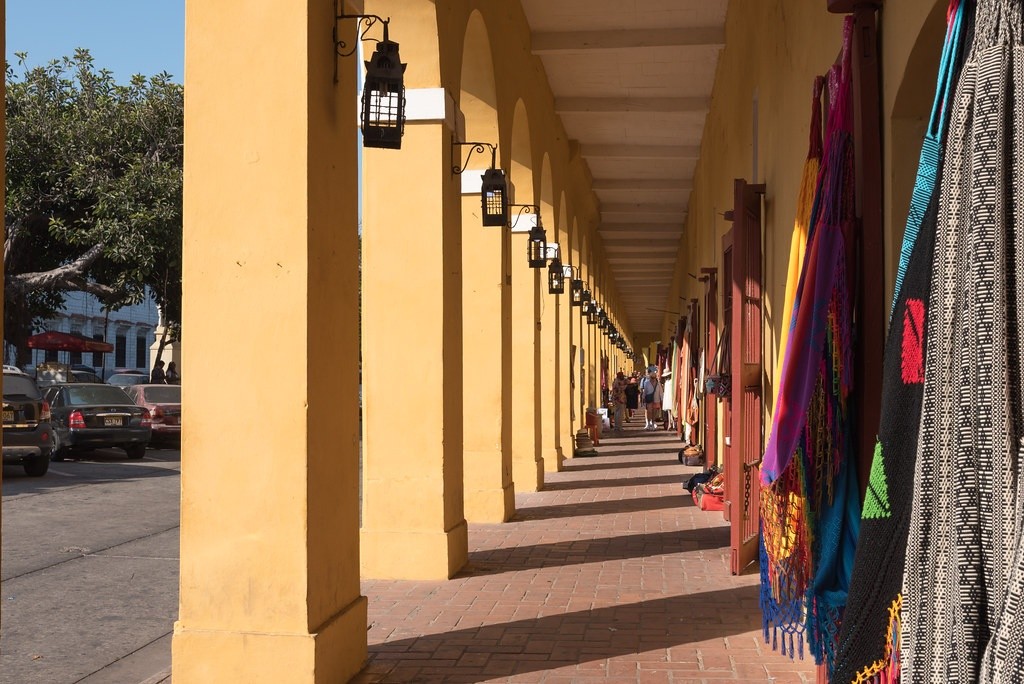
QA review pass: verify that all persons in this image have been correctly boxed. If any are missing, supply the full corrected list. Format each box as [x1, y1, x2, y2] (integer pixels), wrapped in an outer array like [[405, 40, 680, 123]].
[[612, 363, 674, 432], [151, 360, 178, 384]]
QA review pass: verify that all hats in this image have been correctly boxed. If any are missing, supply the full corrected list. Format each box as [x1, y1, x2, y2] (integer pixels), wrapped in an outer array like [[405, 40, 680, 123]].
[[650, 371, 657, 377], [631, 378, 636, 382], [615, 372, 624, 379], [661, 368, 672, 377], [645, 369, 651, 374]]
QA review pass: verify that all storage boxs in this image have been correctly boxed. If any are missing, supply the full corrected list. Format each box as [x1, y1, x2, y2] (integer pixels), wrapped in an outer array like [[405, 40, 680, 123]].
[[602, 418, 611, 432], [597, 408, 609, 418]]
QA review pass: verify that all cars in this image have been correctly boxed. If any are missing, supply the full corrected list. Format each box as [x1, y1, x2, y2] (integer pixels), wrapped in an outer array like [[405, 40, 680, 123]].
[[37, 369, 107, 389], [39, 381, 153, 462], [3, 364, 52, 477], [124, 383, 181, 450], [105, 373, 149, 389]]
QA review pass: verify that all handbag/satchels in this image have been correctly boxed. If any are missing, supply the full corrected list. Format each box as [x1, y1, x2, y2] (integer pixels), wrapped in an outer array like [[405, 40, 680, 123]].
[[645, 392, 654, 403]]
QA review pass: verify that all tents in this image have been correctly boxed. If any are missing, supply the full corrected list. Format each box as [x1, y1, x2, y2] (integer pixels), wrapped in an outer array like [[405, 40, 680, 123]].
[[26, 330, 114, 383]]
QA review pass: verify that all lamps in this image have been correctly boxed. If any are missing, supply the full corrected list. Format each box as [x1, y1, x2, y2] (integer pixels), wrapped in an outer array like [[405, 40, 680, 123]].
[[450, 134, 637, 362], [331, 0, 408, 152]]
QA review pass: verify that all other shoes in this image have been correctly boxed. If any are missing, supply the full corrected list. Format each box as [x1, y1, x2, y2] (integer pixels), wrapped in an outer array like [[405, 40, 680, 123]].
[[625, 419, 630, 423], [614, 428, 624, 433], [644, 426, 650, 430], [651, 426, 656, 430]]
